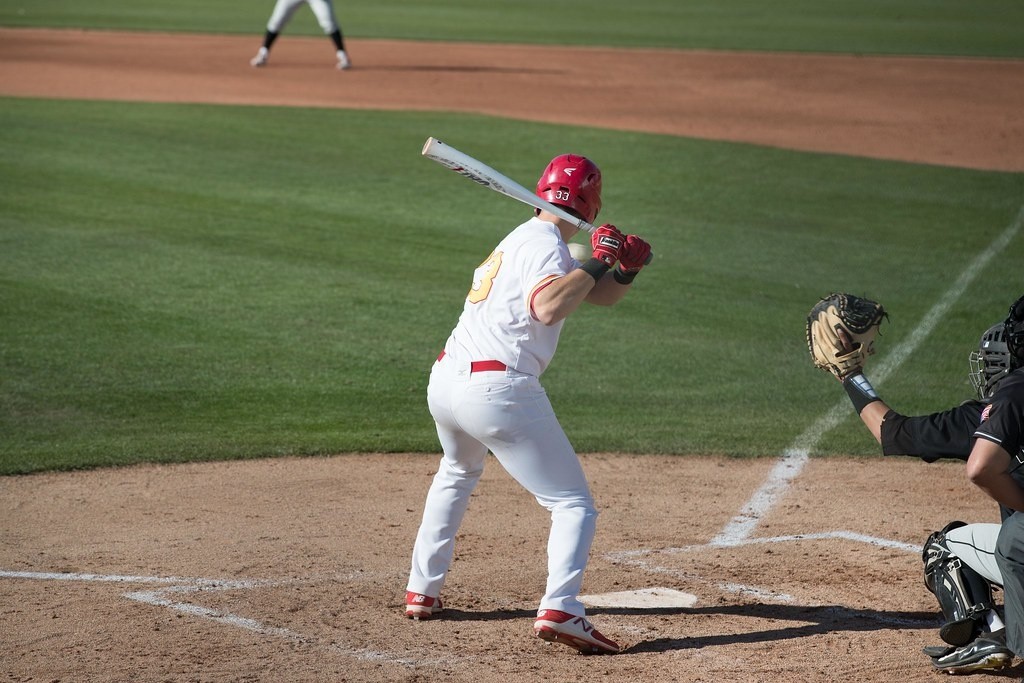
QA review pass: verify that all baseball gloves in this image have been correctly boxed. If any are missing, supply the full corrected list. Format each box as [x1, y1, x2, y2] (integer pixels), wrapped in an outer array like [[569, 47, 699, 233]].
[[805, 291, 891, 381]]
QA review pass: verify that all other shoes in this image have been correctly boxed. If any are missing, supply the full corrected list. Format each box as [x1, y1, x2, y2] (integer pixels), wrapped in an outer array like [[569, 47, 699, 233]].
[[250, 47, 268, 67], [336, 50, 351, 69]]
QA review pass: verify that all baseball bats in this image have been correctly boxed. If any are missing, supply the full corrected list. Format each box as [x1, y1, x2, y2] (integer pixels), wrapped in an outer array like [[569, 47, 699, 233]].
[[421, 135, 654, 266]]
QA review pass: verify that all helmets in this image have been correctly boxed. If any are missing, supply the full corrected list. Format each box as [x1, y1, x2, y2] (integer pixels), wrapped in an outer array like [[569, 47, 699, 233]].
[[533, 154, 602, 225], [1004, 293, 1024, 366], [979, 322, 1013, 393]]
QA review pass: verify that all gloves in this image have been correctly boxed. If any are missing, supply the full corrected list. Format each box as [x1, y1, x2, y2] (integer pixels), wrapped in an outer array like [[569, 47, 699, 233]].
[[590, 225, 626, 268], [621, 234, 651, 273]]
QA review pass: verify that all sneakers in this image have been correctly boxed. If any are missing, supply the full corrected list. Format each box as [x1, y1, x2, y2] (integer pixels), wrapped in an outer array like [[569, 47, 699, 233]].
[[534, 609, 619, 654], [932, 627, 1014, 671], [405, 591, 443, 616]]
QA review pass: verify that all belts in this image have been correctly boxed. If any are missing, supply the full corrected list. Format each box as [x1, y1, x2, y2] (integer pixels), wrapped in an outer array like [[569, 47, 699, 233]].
[[434, 352, 506, 373]]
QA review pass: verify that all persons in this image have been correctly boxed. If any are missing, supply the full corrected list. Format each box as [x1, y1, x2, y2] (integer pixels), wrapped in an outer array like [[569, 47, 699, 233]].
[[248, 1, 353, 70], [805, 294, 1016, 674], [964, 376, 1023, 659], [403, 153, 654, 655]]
